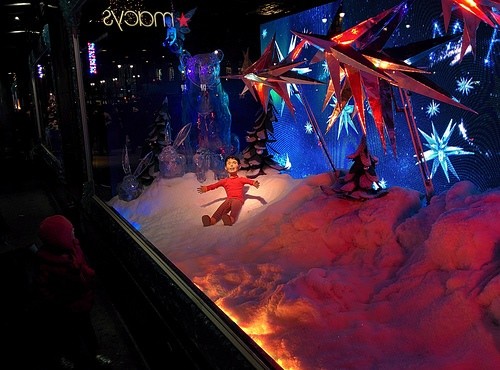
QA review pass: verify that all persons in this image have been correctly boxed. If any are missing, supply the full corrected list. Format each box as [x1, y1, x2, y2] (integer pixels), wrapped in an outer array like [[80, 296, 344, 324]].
[[1, 197, 115, 369], [197, 155, 261, 228]]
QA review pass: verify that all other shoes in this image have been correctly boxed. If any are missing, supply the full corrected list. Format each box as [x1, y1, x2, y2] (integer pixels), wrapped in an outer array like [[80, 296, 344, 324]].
[[61, 357, 74, 368], [95, 354, 113, 367]]
